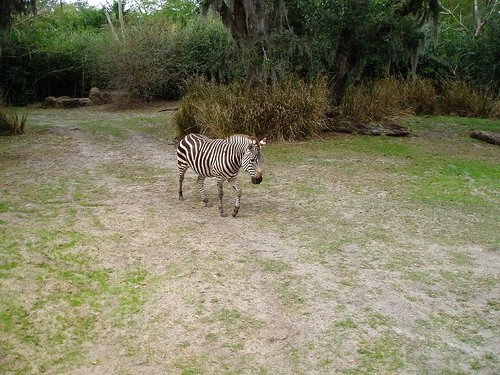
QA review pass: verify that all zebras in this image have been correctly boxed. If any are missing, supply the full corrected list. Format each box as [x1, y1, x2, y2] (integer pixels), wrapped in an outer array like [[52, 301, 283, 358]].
[[175, 132, 264, 218]]
